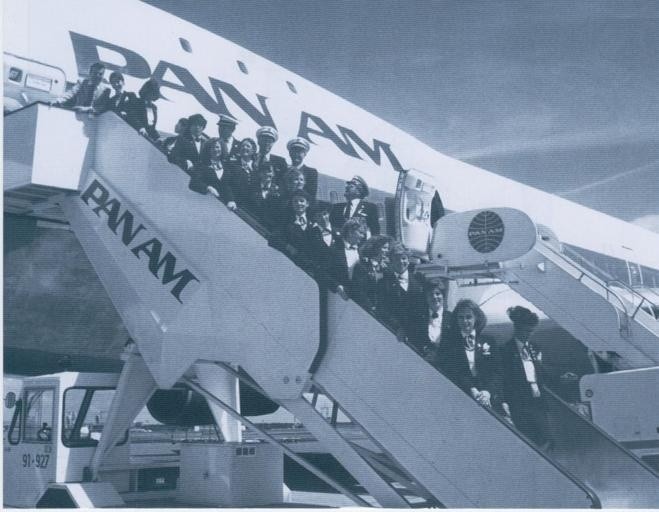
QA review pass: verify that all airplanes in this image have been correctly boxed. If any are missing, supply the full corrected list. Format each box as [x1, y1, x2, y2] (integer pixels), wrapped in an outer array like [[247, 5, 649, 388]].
[[3, 0, 659, 508]]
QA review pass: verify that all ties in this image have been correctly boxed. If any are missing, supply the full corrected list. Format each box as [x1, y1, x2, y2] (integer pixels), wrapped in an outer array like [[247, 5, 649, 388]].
[[344, 202, 352, 219]]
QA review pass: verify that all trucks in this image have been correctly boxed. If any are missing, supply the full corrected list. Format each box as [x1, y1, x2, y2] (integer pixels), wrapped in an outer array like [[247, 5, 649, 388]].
[[4, 371, 284, 507]]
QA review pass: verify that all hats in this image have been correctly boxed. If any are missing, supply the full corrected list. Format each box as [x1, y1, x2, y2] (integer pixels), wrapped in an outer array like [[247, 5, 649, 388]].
[[256, 125, 279, 143], [216, 113, 239, 125], [286, 138, 310, 152], [346, 174, 370, 198]]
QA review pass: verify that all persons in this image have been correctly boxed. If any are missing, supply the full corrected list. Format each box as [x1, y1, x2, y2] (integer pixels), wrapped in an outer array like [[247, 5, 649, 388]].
[[334, 176, 380, 237], [163, 114, 337, 268], [328, 220, 494, 412], [51, 63, 162, 140], [496, 307, 552, 448]]
